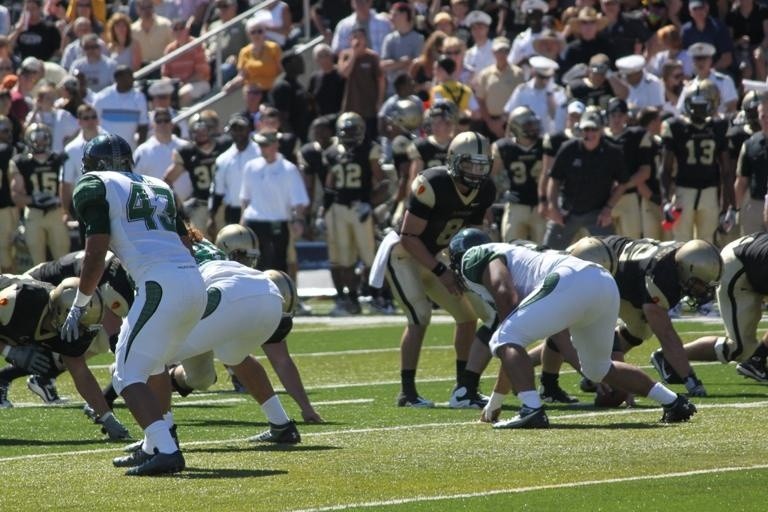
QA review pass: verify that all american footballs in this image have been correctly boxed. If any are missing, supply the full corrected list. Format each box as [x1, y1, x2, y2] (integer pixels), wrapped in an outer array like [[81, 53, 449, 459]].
[[595, 388, 626, 408]]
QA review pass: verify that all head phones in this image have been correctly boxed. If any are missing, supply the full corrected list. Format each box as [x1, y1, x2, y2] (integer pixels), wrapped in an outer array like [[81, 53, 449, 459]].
[[573, 118, 581, 134]]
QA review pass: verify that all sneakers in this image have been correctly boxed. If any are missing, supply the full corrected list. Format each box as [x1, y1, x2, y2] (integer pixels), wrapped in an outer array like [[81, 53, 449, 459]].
[[659, 392, 697, 423], [538, 384, 579, 403], [0, 384, 13, 408], [449, 386, 491, 409], [247, 419, 300, 443], [649, 349, 677, 383], [113, 423, 185, 475], [398, 392, 434, 408], [27, 374, 67, 403], [491, 404, 549, 428], [736, 353, 768, 381]]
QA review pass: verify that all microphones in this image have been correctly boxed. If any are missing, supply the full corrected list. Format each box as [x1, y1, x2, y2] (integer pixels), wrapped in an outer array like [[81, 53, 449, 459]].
[[580, 134, 591, 141]]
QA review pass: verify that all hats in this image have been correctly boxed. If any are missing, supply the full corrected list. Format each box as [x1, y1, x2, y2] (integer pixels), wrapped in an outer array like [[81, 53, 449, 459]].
[[148, 79, 174, 96], [529, 56, 559, 76], [607, 99, 627, 113], [615, 54, 646, 75], [568, 101, 585, 114], [464, 10, 493, 28], [578, 111, 601, 129], [687, 42, 716, 57]]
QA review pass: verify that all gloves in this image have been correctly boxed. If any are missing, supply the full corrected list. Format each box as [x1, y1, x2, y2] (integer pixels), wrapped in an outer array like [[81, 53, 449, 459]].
[[104, 415, 128, 437], [60, 302, 87, 342], [480, 402, 502, 422], [683, 375, 705, 396]]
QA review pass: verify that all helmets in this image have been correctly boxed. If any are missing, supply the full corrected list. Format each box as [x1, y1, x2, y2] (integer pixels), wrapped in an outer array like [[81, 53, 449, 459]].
[[187, 109, 221, 140], [23, 122, 53, 153], [448, 228, 492, 288], [506, 106, 541, 140], [684, 79, 721, 115], [215, 224, 260, 268], [569, 236, 617, 276], [335, 111, 365, 146], [264, 269, 298, 318], [675, 237, 723, 309], [48, 276, 105, 337], [446, 131, 494, 188], [81, 133, 134, 174]]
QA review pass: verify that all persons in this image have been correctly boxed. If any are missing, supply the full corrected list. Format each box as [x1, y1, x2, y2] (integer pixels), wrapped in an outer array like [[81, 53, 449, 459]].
[[649, 231, 768, 385], [368, 132, 496, 409], [448, 228, 697, 430], [62, 132, 208, 476], [449, 238, 636, 410], [1, 1, 768, 318], [1, 219, 326, 444], [512, 235, 725, 397]]
